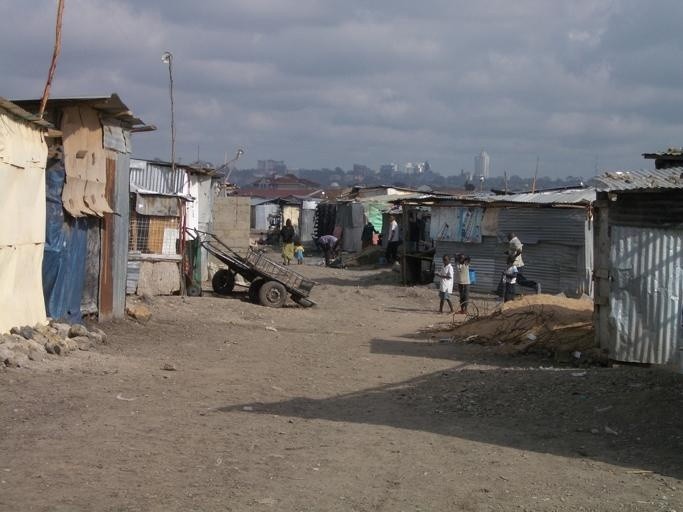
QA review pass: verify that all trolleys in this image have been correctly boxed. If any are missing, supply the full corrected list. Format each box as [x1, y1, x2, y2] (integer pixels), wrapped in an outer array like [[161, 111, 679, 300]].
[[180, 225, 317, 309]]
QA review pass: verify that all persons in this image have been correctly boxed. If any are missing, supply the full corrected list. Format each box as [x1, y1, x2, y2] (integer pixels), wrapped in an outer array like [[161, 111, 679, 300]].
[[457, 255, 471, 314], [434, 255, 454, 315], [504, 233, 541, 303], [280, 219, 295, 266], [317, 235, 339, 258], [295, 242, 304, 265], [385, 215, 399, 263], [361, 220, 378, 250]]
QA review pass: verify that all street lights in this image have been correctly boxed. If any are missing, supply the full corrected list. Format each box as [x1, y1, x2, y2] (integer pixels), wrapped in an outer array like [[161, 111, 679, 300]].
[[161, 47, 177, 195]]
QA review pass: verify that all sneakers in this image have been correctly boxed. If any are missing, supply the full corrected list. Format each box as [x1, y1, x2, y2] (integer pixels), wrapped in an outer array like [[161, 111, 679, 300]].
[[535, 283, 541, 295]]
